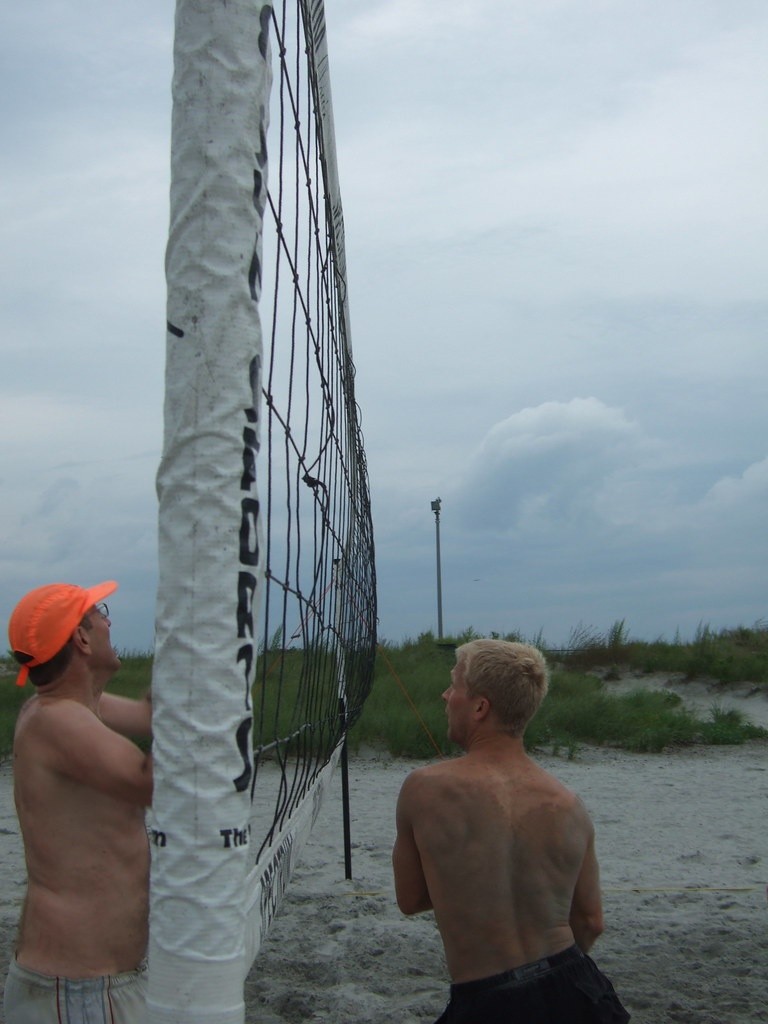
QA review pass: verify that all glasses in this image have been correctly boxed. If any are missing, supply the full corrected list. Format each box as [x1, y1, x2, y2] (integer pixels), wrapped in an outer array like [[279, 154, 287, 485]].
[[81, 602, 109, 621]]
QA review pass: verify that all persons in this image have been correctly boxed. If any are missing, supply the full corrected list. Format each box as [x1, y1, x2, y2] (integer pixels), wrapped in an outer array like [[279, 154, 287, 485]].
[[3, 582, 153, 1024], [390, 639, 631, 1024]]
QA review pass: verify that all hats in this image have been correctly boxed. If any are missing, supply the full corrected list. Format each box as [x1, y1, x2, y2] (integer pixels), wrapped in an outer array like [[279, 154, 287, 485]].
[[9, 581, 118, 687]]
